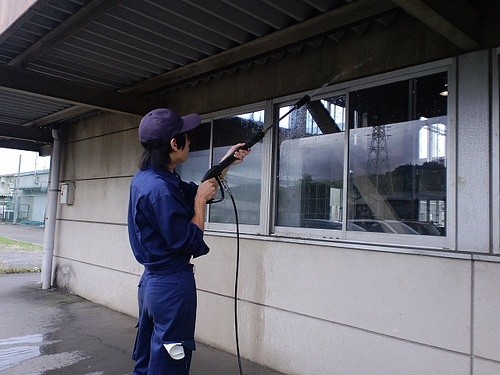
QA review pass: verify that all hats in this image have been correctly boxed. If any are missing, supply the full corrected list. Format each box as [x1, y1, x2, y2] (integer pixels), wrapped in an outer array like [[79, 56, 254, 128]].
[[138, 108, 201, 145]]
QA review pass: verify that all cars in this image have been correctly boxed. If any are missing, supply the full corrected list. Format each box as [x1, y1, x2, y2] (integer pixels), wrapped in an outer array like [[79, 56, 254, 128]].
[[300, 217, 442, 237]]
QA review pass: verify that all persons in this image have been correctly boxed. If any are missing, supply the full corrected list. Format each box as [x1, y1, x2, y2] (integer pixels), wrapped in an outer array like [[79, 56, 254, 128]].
[[130, 109, 251, 375]]
